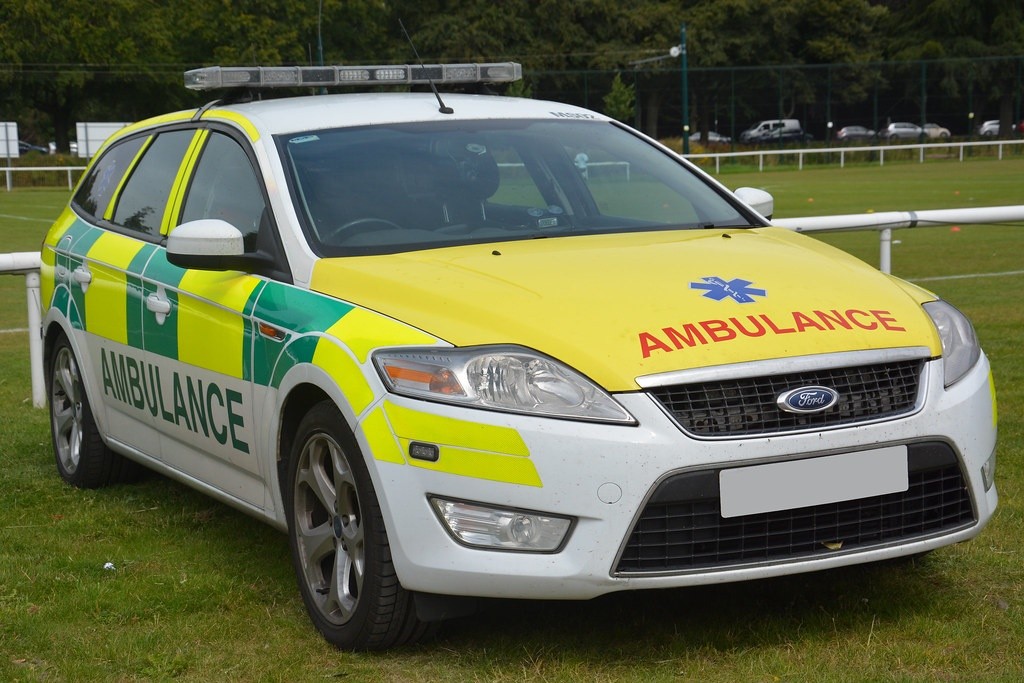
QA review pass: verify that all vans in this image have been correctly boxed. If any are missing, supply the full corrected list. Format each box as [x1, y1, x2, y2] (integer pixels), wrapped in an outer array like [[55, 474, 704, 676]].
[[739, 118, 802, 145]]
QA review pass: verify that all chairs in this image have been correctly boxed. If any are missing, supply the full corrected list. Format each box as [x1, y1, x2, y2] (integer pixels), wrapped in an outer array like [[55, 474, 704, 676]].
[[434, 149, 522, 233]]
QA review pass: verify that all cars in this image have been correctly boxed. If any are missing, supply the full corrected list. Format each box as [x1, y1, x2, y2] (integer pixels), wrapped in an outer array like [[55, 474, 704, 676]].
[[690, 130, 732, 146], [18, 141, 50, 156], [836, 125, 883, 140], [748, 129, 815, 146], [974, 118, 1018, 138], [878, 121, 931, 144], [922, 122, 953, 141]]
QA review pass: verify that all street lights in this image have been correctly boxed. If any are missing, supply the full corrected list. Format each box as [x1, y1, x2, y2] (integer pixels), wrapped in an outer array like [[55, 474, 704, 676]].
[[671, 26, 689, 160]]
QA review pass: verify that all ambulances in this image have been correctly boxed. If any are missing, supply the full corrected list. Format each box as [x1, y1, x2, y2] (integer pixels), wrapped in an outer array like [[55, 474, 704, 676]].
[[38, 64, 1000, 659]]
